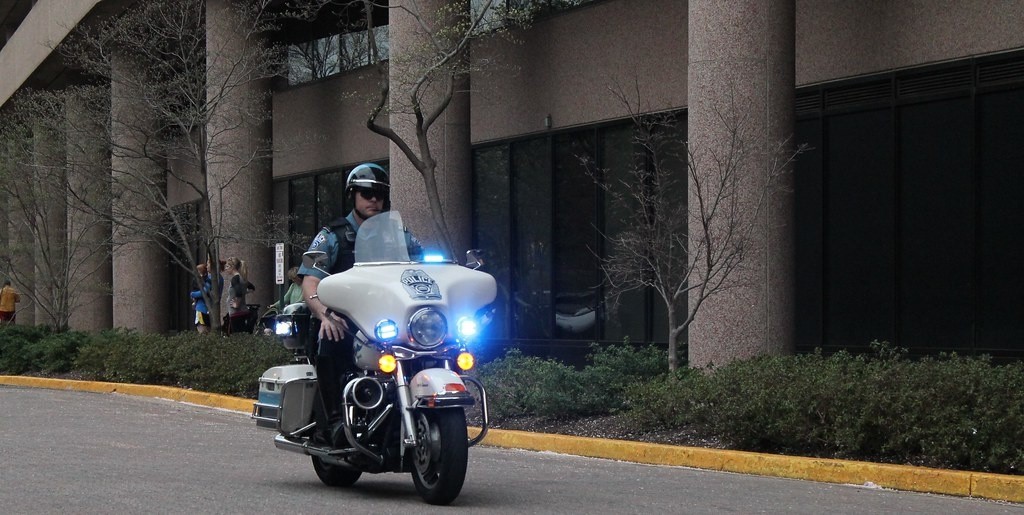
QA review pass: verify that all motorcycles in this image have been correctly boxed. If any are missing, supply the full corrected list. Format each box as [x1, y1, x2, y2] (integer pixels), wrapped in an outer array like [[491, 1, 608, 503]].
[[250, 209, 497, 503]]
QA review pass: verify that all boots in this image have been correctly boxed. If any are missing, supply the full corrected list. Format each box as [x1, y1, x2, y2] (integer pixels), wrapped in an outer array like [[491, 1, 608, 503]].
[[312, 354, 348, 445]]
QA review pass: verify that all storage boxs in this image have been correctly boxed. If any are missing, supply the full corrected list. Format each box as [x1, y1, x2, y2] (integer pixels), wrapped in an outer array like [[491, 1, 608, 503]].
[[256, 364, 317, 431]]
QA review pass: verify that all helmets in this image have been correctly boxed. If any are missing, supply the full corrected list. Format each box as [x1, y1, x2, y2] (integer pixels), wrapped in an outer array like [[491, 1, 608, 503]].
[[344, 162, 391, 193]]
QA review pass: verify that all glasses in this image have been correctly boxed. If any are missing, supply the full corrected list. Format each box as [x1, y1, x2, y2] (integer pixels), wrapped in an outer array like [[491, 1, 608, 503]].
[[356, 188, 386, 201]]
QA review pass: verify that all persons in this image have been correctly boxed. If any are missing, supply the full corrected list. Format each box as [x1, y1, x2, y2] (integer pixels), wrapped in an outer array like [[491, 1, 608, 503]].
[[190, 256, 255, 333], [296, 163, 426, 448], [267, 267, 305, 311], [0, 279, 20, 325]]
